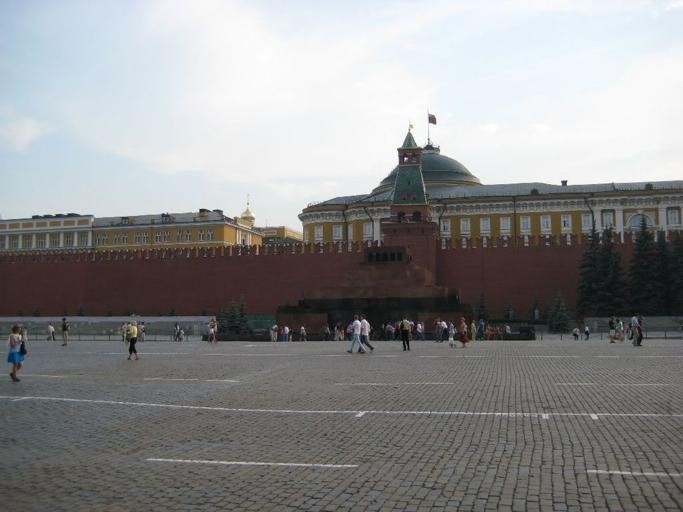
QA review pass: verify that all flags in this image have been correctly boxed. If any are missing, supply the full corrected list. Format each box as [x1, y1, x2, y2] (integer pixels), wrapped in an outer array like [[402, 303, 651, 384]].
[[429, 114, 436, 124]]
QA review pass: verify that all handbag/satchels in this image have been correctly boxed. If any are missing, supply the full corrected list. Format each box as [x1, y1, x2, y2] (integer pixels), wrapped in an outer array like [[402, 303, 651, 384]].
[[20, 341, 27, 355]]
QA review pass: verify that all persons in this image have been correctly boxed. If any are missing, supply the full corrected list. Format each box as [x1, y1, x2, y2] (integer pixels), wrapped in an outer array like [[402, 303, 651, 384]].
[[174, 321, 180, 341], [120, 319, 146, 360], [178, 329, 184, 342], [7, 324, 25, 382], [272, 310, 513, 353], [572, 327, 581, 340], [207, 325, 215, 344], [52, 323, 56, 340], [609, 311, 643, 347], [210, 317, 217, 343], [61, 317, 69, 347], [585, 323, 590, 340], [47, 322, 52, 341]]
